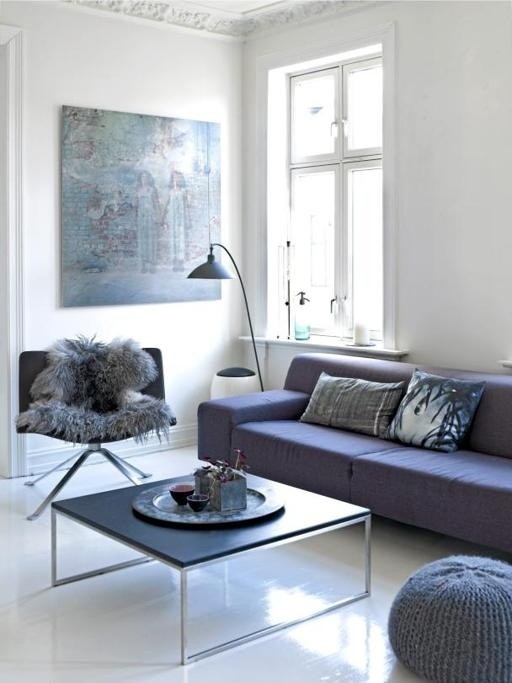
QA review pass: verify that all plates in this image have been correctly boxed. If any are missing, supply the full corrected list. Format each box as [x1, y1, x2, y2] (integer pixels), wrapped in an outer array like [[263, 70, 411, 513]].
[[132, 481, 284, 525]]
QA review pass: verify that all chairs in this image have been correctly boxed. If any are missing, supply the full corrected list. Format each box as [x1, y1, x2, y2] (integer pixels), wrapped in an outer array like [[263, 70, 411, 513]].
[[15, 333, 176, 521]]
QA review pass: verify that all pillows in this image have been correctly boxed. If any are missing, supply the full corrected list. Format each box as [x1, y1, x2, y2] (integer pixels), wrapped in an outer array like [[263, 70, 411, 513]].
[[380, 368, 487, 453], [300, 371, 404, 438]]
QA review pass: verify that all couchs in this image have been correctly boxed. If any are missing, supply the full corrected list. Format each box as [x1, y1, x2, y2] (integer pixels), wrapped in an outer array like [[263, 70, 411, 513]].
[[199, 353, 512, 557]]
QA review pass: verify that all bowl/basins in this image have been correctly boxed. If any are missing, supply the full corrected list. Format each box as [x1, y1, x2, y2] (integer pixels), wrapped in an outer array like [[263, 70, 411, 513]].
[[168, 484, 194, 505], [187, 494, 209, 512]]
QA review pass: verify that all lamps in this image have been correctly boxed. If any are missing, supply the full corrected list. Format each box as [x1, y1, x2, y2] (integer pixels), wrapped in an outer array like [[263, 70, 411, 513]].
[[186, 244, 263, 390]]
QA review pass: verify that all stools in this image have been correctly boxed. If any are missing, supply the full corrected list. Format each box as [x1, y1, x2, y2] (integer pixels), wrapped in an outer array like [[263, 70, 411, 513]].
[[390, 557, 512, 681], [212, 368, 261, 398]]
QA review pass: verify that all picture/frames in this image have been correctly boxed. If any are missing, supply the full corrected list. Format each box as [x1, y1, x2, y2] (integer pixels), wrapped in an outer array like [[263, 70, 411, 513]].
[[60, 105, 222, 308]]
